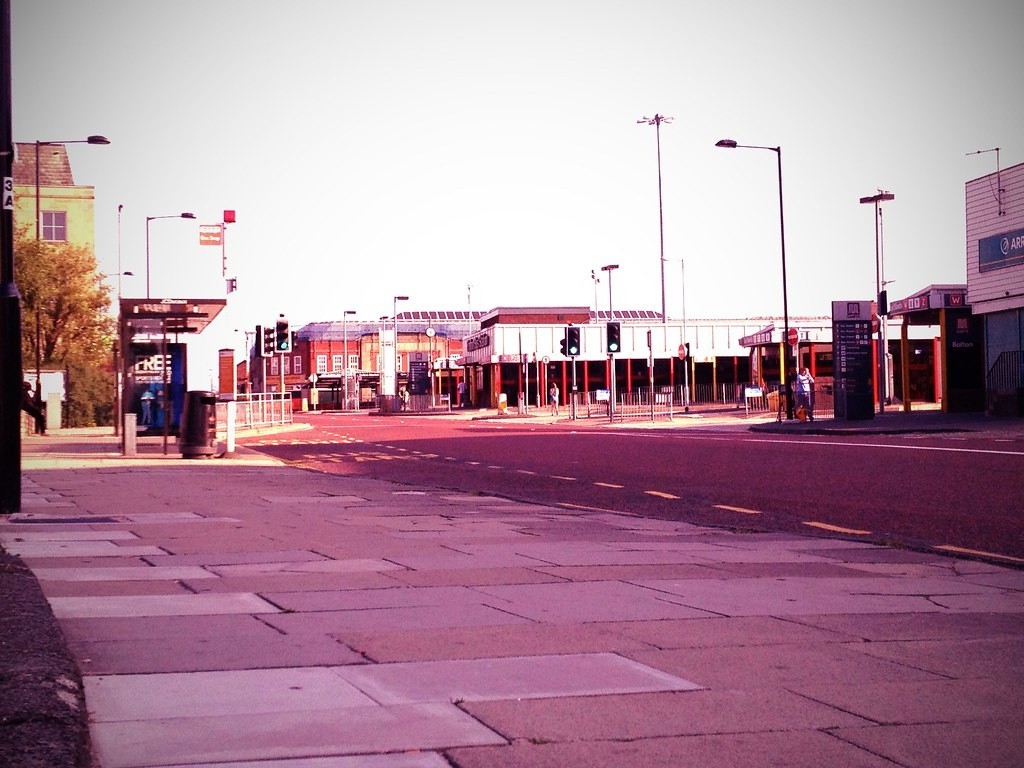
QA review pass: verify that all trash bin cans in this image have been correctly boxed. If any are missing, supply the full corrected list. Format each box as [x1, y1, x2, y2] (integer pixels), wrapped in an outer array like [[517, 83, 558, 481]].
[[517, 391, 525, 414], [536, 395, 540, 408], [179, 390, 217, 460]]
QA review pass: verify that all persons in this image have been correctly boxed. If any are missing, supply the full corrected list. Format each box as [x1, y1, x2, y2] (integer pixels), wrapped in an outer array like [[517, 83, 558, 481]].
[[457, 379, 468, 408], [398, 387, 409, 411], [549, 382, 559, 416], [795, 367, 815, 424], [21, 381, 51, 437]]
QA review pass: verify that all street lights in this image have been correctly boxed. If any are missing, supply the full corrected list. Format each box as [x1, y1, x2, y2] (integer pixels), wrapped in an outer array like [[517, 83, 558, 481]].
[[35, 134, 112, 433], [715, 139, 789, 413], [393, 295, 409, 412], [118, 203, 124, 299], [466, 282, 471, 334], [591, 270, 598, 314], [234, 329, 255, 396], [146, 211, 197, 302], [636, 114, 675, 325], [601, 265, 620, 320], [97, 269, 135, 280], [343, 311, 356, 412], [860, 191, 895, 411]]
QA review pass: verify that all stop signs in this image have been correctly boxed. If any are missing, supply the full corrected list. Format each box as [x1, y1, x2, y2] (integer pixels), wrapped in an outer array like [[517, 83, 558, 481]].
[[678, 344, 685, 361], [787, 327, 799, 347]]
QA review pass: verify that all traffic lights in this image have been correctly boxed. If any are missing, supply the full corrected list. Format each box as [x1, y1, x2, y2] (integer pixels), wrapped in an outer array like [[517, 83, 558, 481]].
[[566, 326, 580, 357], [275, 320, 291, 353], [260, 326, 276, 357], [607, 323, 621, 354]]
